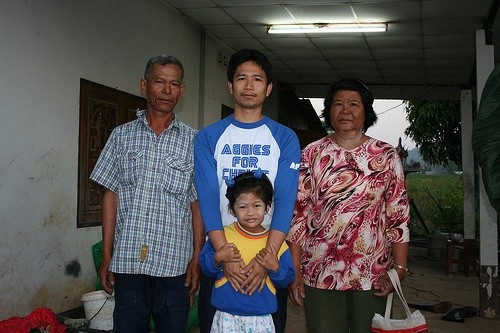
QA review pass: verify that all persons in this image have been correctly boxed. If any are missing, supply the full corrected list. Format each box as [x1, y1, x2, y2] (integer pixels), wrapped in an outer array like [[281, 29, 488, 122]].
[[89, 54, 207, 333], [198, 168, 295, 333], [286, 76, 410, 333], [193, 48, 301, 333]]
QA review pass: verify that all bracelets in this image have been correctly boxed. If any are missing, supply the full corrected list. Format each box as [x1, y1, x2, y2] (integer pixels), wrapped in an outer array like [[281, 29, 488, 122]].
[[392, 263, 408, 274]]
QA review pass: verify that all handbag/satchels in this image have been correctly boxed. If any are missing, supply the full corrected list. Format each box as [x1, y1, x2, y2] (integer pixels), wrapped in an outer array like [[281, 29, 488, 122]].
[[370, 268, 428, 333]]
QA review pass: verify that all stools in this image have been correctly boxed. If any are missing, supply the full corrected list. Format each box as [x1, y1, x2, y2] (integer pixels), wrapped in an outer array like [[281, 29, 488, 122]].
[[445, 240, 469, 278]]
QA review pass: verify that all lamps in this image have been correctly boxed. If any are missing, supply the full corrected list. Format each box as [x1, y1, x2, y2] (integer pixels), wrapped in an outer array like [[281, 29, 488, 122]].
[[266, 23, 388, 34]]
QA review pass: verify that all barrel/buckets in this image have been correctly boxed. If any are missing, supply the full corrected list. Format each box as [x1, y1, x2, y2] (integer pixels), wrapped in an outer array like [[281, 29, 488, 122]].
[[427, 231, 466, 275], [80, 288, 116, 331]]
